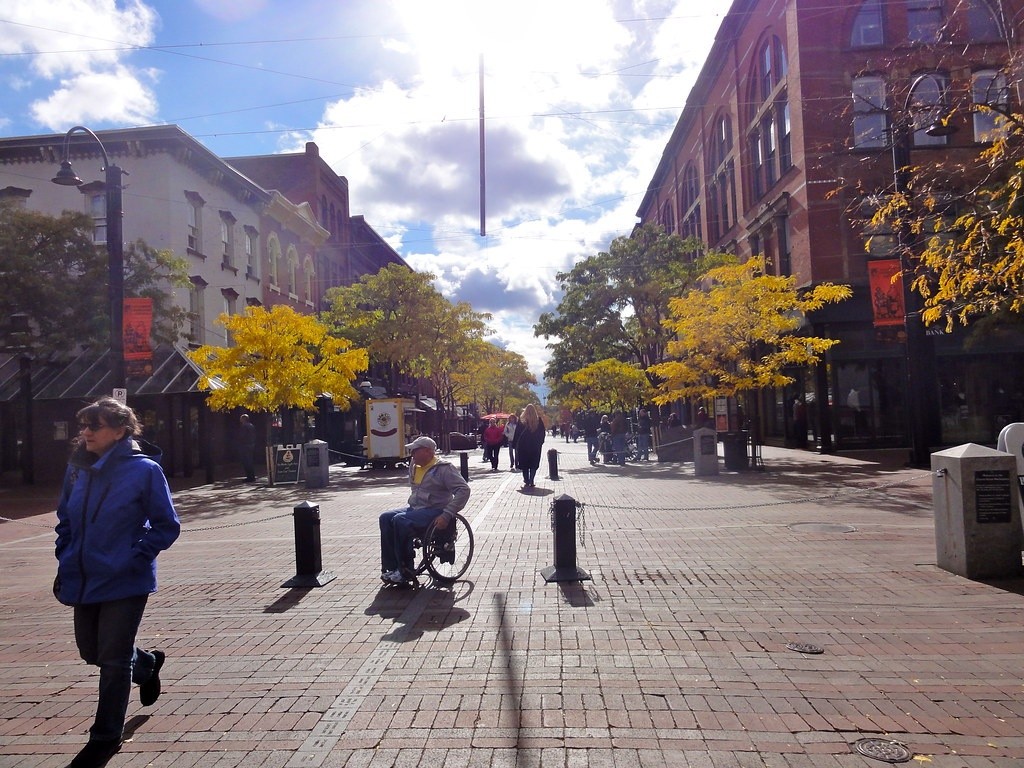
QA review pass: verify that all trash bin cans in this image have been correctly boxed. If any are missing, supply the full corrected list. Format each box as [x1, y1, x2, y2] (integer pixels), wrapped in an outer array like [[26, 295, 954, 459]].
[[723, 431, 748, 471]]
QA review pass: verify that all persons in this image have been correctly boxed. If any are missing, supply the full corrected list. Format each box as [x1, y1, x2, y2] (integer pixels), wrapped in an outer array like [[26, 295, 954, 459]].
[[236, 414, 255, 481], [379, 437, 470, 583], [612, 409, 628, 465], [632, 409, 649, 462], [585, 410, 599, 464], [792, 392, 807, 447], [501, 414, 519, 472], [53, 398, 180, 768], [552, 423, 557, 436], [600, 415, 612, 461], [513, 404, 545, 486], [698, 407, 706, 422], [671, 414, 682, 428], [560, 421, 578, 442], [478, 417, 502, 471]]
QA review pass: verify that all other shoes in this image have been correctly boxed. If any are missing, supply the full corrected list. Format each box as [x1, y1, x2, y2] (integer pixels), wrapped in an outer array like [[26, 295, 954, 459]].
[[70, 733, 124, 768], [140, 650, 165, 706]]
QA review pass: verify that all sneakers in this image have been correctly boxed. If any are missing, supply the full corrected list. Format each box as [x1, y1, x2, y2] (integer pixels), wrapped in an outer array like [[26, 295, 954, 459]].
[[387, 570, 408, 583], [380, 571, 394, 580]]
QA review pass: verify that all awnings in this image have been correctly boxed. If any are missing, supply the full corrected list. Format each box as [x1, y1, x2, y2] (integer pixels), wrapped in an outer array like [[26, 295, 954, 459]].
[[362, 384, 425, 413], [412, 394, 474, 420]]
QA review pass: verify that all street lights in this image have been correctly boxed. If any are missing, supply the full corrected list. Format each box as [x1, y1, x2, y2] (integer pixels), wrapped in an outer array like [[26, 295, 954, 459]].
[[50, 125, 127, 392], [890, 72, 959, 461]]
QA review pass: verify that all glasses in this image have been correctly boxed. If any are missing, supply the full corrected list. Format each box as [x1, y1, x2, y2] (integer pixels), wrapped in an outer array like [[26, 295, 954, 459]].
[[78, 422, 127, 432]]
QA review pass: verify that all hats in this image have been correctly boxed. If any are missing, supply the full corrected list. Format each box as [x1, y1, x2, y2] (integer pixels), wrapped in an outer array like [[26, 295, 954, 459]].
[[404, 437, 437, 451]]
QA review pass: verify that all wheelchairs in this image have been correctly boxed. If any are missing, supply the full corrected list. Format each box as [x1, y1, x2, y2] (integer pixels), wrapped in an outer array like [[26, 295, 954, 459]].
[[383, 513, 475, 592]]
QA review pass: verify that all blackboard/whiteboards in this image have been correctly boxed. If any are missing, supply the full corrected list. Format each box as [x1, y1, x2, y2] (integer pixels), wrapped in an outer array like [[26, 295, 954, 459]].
[[274, 448, 305, 485]]
